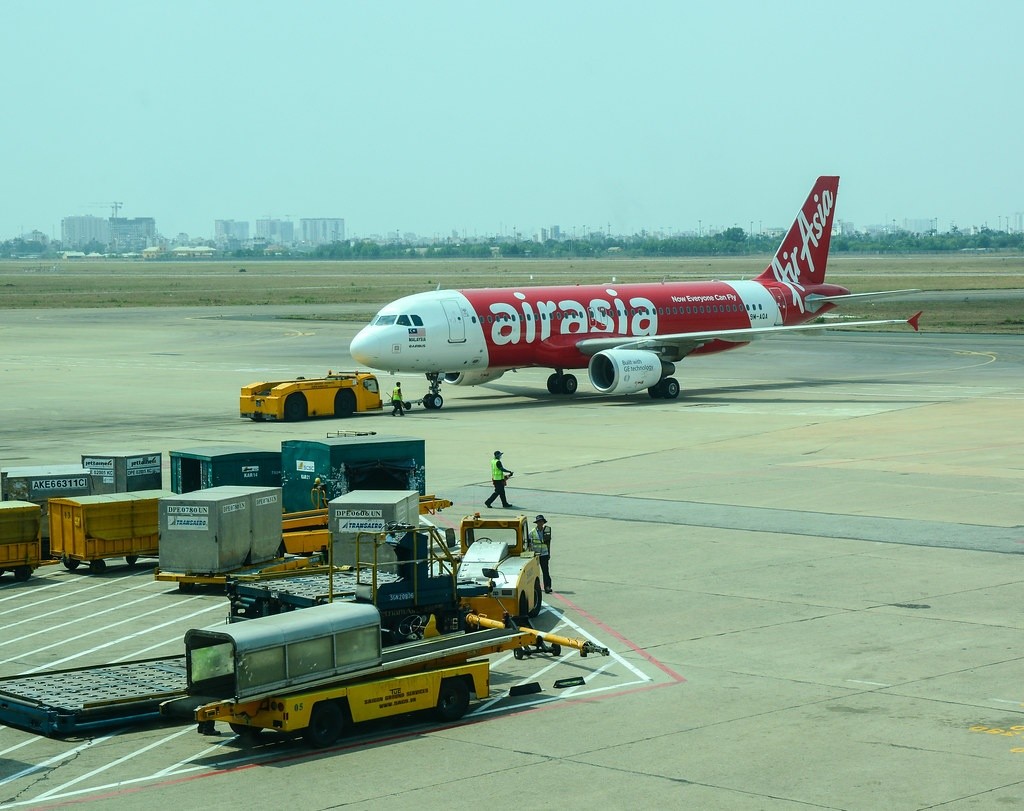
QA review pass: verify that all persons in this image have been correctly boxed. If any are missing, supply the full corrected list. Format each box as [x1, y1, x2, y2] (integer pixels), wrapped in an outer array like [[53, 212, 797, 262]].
[[389, 382, 406, 417], [528, 515, 553, 594], [484, 451, 513, 508]]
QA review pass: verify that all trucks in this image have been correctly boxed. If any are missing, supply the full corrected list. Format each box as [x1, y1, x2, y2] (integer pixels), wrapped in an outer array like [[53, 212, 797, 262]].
[[239, 371, 385, 423]]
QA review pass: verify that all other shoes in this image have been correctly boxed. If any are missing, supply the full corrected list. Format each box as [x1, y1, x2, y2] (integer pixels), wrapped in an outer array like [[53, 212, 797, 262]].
[[400, 413, 404, 415], [485, 502, 492, 508], [503, 503, 512, 507], [391, 413, 395, 416], [545, 586, 553, 593]]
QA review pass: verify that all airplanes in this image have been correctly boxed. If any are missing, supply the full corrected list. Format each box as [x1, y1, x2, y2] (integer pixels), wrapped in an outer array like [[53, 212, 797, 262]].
[[348, 174, 925, 399]]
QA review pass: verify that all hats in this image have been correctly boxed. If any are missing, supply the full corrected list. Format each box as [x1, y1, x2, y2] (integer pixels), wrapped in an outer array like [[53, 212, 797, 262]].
[[533, 515, 547, 523], [494, 451, 503, 458]]
